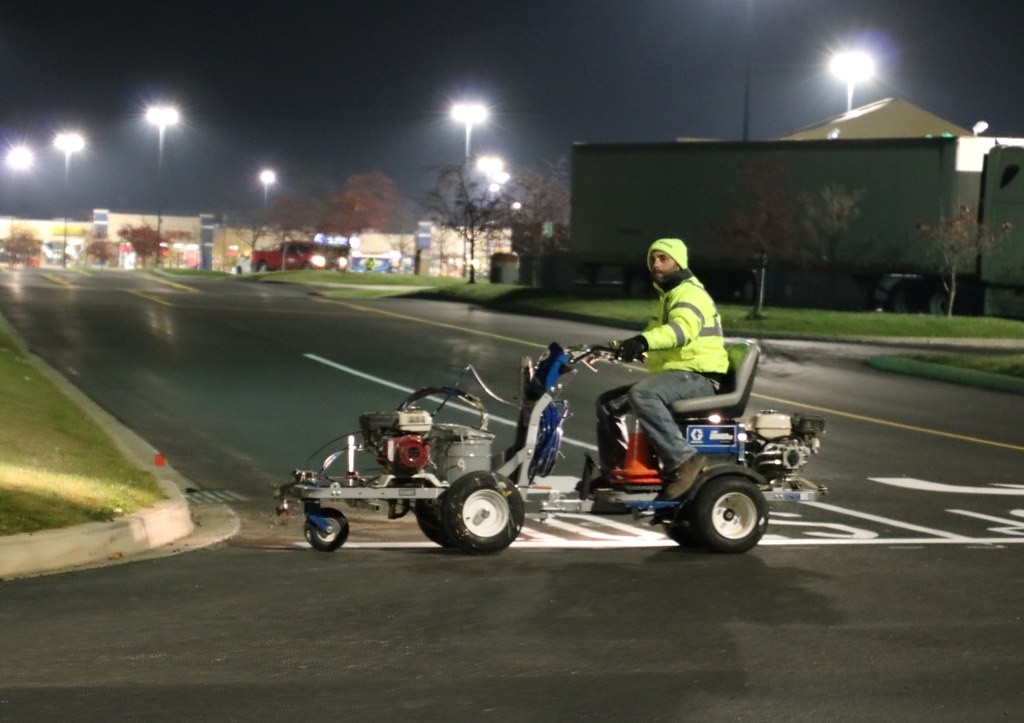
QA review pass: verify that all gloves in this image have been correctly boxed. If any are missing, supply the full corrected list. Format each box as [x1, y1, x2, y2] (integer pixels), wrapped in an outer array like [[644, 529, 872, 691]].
[[616, 335, 648, 363]]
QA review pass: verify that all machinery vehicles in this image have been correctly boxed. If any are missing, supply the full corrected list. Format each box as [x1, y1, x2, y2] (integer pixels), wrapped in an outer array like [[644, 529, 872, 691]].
[[273, 338, 827, 557]]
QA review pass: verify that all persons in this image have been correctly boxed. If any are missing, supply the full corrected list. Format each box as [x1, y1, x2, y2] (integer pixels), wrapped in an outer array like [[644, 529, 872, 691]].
[[575, 236, 729, 499]]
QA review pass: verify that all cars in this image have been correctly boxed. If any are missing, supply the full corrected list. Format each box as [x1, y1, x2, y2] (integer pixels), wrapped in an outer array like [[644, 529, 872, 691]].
[[251, 237, 350, 276]]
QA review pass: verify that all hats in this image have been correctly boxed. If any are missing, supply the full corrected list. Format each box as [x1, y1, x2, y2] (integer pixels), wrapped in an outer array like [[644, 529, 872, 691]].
[[647, 238, 688, 273]]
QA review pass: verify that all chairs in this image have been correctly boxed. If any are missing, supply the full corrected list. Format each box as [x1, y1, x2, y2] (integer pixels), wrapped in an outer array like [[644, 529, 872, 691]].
[[674, 338, 763, 416]]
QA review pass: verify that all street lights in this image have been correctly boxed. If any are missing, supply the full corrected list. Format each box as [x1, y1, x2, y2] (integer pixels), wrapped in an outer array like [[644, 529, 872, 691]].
[[52, 131, 85, 264], [144, 104, 180, 267], [446, 99, 490, 275]]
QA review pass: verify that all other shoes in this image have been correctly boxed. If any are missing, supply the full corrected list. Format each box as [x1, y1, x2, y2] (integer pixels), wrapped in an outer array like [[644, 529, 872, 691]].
[[654, 453, 707, 501]]
